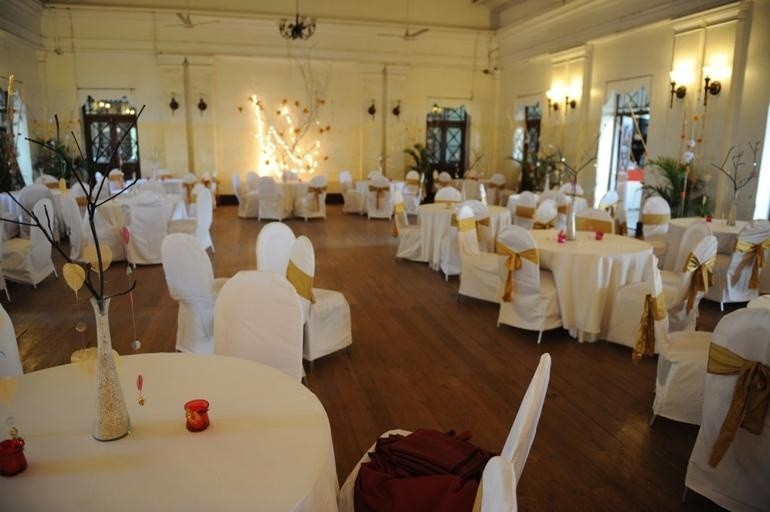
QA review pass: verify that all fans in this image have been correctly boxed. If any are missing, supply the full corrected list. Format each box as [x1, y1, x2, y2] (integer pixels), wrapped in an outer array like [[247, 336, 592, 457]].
[[163, 0, 220, 29], [375, 0, 431, 43]]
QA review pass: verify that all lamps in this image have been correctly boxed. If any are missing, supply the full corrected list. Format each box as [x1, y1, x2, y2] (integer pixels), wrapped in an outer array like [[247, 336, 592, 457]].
[[391, 99, 402, 122], [197, 97, 207, 117], [168, 91, 180, 116], [545, 90, 560, 120], [562, 89, 578, 117], [667, 70, 687, 109], [368, 97, 378, 122], [700, 65, 723, 108]]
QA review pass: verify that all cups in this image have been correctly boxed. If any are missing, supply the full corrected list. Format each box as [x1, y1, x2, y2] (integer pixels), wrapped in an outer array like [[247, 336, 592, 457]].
[[184, 399, 209, 432], [0, 439, 27, 476]]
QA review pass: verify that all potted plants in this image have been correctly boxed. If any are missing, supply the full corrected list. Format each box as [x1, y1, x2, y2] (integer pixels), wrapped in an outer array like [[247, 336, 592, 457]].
[[710, 138, 762, 227], [546, 134, 603, 243], [0, 103, 157, 445]]
